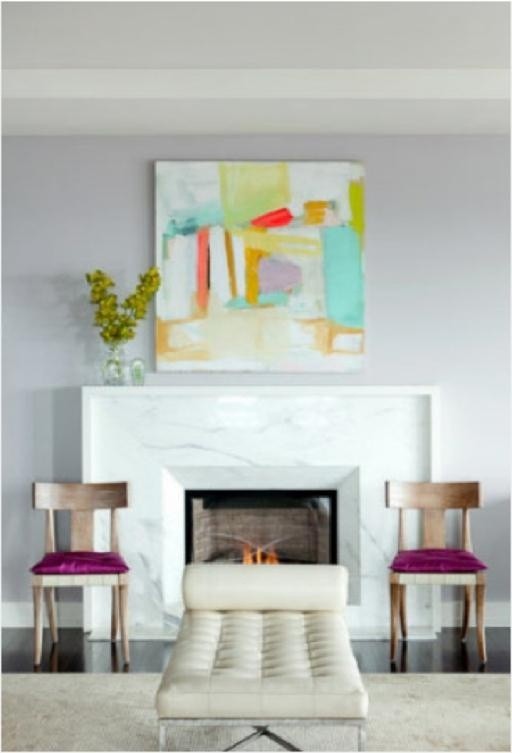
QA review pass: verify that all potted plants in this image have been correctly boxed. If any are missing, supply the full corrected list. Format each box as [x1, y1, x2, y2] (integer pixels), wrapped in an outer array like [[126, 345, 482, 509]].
[[85, 266, 163, 384]]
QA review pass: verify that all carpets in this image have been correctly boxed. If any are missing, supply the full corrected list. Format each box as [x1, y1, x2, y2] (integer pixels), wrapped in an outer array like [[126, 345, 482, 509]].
[[2, 671, 509, 750]]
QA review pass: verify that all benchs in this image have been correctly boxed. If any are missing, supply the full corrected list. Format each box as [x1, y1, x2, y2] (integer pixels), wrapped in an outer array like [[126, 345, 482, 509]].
[[155, 562, 369, 749]]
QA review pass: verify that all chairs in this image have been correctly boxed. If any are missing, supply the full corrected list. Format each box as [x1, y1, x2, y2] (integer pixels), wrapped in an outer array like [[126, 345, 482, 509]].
[[382, 481, 491, 667], [34, 483, 135, 665]]
